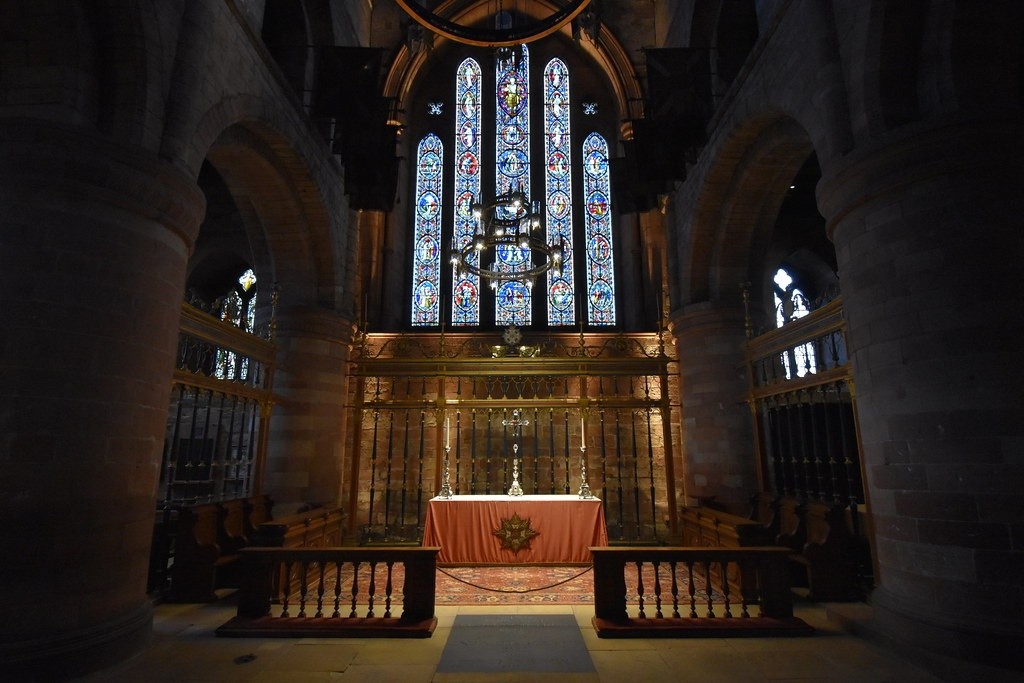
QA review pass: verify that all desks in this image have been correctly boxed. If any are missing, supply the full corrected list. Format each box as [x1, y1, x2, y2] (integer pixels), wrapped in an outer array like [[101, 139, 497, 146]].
[[422, 494, 607, 566]]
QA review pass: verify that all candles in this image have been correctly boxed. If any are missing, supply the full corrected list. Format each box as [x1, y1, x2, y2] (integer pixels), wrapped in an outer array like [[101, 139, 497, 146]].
[[582, 417, 586, 447], [446, 416, 450, 446]]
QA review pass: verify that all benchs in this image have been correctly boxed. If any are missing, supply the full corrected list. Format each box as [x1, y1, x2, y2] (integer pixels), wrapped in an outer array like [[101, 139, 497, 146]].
[[175, 494, 282, 604], [701, 491, 846, 598]]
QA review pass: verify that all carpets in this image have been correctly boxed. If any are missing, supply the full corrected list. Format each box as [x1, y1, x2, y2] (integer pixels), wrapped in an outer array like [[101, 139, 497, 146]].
[[434, 613, 599, 675]]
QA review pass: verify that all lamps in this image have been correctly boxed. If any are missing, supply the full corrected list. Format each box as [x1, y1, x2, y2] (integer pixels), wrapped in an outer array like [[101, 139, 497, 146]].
[[450, 71, 564, 291]]
